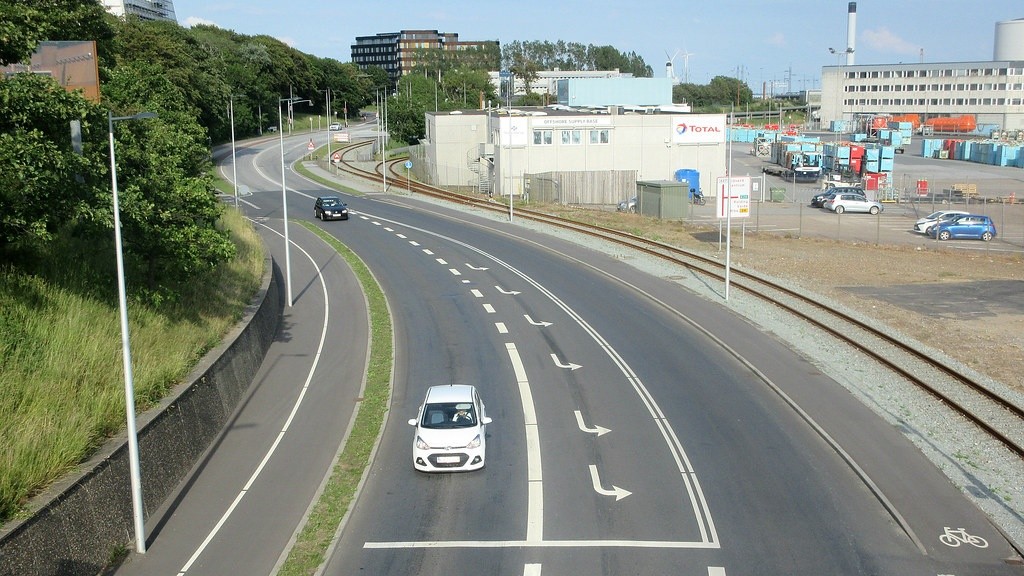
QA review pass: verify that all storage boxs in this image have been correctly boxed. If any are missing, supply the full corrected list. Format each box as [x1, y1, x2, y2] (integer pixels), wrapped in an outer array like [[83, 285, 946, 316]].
[[726, 123, 1024, 195]]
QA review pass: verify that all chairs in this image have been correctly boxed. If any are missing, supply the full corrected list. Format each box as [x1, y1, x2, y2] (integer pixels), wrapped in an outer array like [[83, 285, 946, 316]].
[[428, 403, 448, 425]]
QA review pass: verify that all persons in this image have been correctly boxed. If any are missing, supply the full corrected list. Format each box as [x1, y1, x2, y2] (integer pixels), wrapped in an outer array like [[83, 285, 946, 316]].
[[331, 200, 338, 206], [364, 116, 366, 123], [452, 409, 473, 423]]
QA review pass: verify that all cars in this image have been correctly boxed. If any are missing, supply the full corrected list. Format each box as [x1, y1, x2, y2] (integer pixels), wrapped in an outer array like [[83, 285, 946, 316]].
[[313, 196, 350, 221], [617, 195, 636, 212], [329, 122, 343, 131], [409, 384, 494, 474], [811, 187, 884, 215], [914, 210, 997, 241]]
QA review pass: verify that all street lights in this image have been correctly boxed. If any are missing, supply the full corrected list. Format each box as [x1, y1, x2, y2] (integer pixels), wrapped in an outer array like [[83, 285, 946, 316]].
[[108, 109, 160, 553], [287, 100, 314, 135], [278, 96, 303, 307], [230, 93, 246, 206]]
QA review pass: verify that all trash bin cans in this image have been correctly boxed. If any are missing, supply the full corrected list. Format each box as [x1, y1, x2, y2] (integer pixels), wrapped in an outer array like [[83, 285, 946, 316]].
[[770, 187, 786, 201], [269, 126, 277, 133], [676, 169, 699, 197]]
